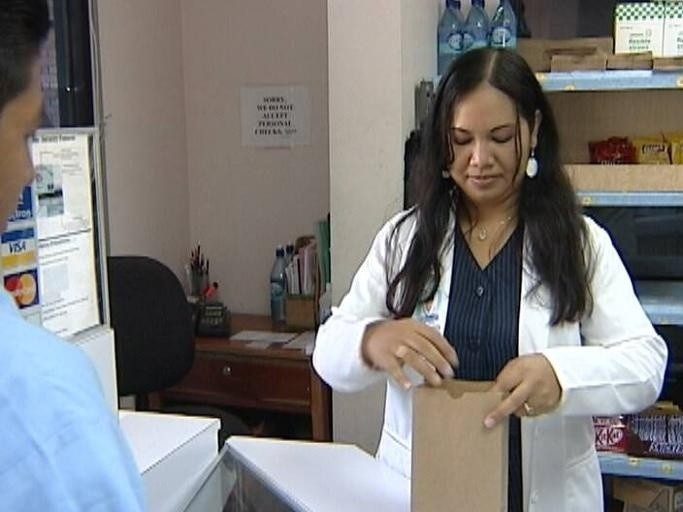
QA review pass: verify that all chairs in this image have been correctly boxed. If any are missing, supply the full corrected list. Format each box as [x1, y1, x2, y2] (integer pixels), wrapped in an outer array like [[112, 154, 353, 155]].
[[105, 256, 252, 449]]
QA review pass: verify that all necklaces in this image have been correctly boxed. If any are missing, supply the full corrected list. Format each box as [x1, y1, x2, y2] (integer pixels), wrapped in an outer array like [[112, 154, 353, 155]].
[[472, 215, 511, 241]]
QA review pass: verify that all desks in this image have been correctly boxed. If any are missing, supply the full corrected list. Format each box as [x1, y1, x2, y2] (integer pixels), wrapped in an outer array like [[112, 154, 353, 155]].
[[147, 311, 333, 443]]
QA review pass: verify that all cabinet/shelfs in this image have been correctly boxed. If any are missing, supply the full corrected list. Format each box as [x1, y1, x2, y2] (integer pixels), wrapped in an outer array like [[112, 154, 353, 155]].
[[431, 67, 683, 511]]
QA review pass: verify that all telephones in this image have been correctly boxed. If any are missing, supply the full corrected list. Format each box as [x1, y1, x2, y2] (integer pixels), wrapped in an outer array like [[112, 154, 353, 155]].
[[192, 303, 232, 337]]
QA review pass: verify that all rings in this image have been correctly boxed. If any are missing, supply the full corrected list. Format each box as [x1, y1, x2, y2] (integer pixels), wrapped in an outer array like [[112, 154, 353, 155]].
[[523, 401, 535, 416], [395, 344, 408, 359]]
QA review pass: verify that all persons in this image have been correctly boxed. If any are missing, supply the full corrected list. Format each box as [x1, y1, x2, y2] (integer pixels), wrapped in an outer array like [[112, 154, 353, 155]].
[[0, 0, 150, 511], [311, 45, 669, 512]]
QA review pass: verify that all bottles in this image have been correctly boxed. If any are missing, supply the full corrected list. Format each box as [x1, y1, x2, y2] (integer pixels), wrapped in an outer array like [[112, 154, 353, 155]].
[[461, 0, 491, 56], [437, 0, 464, 76], [489, 0, 518, 53], [269, 241, 295, 333]]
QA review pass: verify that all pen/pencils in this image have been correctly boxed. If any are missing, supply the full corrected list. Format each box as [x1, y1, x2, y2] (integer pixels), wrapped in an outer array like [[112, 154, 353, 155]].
[[190, 241, 209, 275]]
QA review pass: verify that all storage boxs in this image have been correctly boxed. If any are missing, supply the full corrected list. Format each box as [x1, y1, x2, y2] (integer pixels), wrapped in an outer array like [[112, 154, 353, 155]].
[[610, 471, 683, 512]]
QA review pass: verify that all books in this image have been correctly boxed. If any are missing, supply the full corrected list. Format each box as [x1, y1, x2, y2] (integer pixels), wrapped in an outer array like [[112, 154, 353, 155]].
[[606, 50, 653, 71], [649, 56, 682, 72], [549, 52, 607, 76], [543, 43, 606, 56]]
